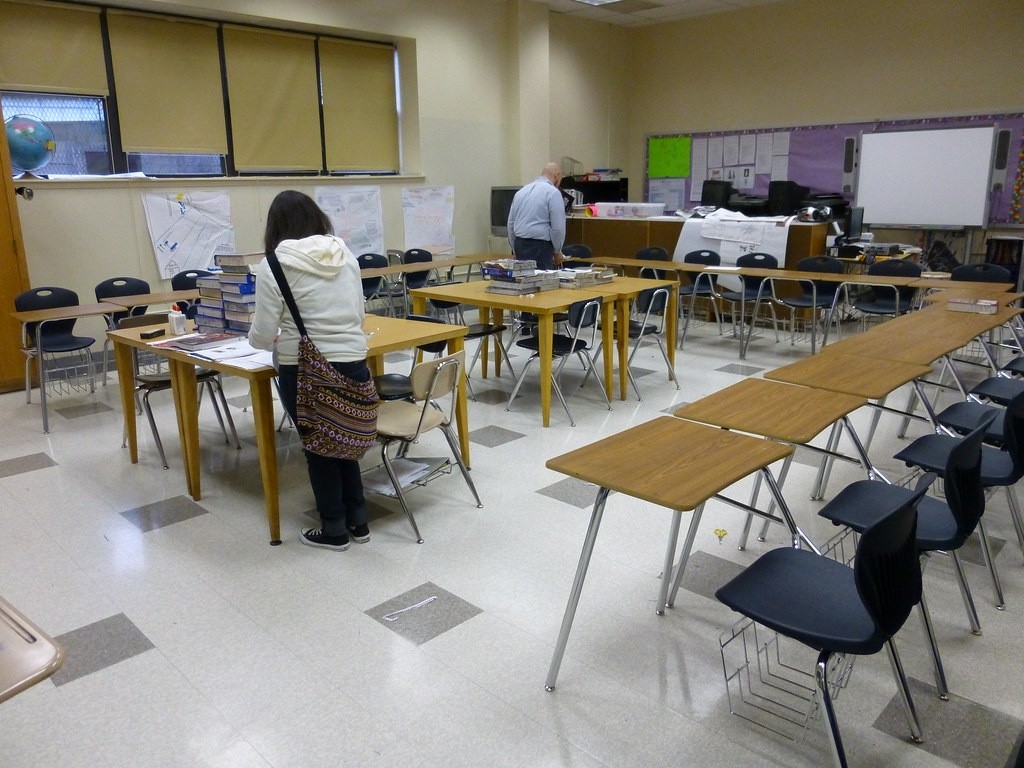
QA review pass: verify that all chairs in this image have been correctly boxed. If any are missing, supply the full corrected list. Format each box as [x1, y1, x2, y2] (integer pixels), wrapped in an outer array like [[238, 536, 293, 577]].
[[15, 240, 1024, 768]]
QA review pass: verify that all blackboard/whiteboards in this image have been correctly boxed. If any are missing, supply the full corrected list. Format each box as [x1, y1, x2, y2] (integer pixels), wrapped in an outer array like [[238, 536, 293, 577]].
[[854, 122, 1000, 230]]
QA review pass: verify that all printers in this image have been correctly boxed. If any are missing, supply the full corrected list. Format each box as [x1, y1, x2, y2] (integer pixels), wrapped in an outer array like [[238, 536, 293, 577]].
[[800, 193, 850, 219], [727, 193, 768, 216]]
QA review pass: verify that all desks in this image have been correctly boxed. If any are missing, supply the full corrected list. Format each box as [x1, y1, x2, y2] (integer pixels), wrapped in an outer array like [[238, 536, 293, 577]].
[[925, 289, 1024, 352], [108, 309, 377, 497], [8, 302, 128, 434], [762, 351, 939, 497], [667, 375, 882, 610], [544, 414, 800, 693], [822, 275, 923, 349], [584, 277, 680, 401], [873, 302, 1002, 379], [98, 290, 200, 388], [910, 278, 1024, 361], [681, 266, 835, 359], [361, 259, 485, 318], [410, 280, 619, 426], [828, 332, 968, 437], [581, 256, 704, 347], [174, 315, 470, 545]]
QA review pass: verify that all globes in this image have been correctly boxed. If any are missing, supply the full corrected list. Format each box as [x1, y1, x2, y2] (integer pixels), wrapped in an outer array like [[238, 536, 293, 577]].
[[4, 114, 55, 180]]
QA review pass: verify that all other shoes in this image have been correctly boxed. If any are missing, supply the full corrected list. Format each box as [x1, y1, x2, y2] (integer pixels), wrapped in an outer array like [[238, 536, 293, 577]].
[[522, 325, 538, 337]]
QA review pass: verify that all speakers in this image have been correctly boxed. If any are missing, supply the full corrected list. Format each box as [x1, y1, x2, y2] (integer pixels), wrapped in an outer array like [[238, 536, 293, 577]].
[[990, 128, 1013, 193], [843, 136, 856, 193]]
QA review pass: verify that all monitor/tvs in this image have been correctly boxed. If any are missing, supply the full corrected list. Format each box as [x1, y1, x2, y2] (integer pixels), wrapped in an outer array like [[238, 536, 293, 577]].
[[701, 181, 738, 210], [490, 186, 524, 237], [767, 180, 810, 217]]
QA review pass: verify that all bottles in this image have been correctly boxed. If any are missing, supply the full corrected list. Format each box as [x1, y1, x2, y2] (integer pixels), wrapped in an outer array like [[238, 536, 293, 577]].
[[169, 304, 188, 335]]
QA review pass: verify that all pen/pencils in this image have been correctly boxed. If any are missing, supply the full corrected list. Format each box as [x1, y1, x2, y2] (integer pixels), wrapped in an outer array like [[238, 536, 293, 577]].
[[561, 269, 575, 272]]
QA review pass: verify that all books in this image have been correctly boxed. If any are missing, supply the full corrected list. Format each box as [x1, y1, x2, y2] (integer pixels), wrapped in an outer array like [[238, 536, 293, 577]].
[[193, 253, 267, 338], [167, 331, 245, 350], [947, 298, 999, 314], [482, 258, 615, 295]]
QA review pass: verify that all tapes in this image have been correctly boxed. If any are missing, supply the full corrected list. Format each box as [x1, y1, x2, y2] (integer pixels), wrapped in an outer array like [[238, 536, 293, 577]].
[[585, 206, 597, 218]]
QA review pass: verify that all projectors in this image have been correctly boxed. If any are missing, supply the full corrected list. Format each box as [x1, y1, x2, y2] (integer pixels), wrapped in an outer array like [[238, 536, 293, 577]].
[[864, 243, 899, 256]]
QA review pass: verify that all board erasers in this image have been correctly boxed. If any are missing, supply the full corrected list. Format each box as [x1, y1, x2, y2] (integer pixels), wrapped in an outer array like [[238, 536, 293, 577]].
[[140, 328, 165, 339]]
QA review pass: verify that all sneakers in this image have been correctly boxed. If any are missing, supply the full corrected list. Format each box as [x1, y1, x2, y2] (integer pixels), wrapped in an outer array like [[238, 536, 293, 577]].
[[346, 517, 371, 542], [299, 528, 350, 551]]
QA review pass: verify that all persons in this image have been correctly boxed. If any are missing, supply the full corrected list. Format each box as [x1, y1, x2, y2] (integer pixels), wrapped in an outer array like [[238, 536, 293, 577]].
[[248, 190, 371, 551], [508, 162, 566, 336]]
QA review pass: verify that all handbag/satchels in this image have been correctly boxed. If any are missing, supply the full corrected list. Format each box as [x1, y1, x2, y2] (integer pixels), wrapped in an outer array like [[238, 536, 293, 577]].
[[297, 334, 380, 462]]
